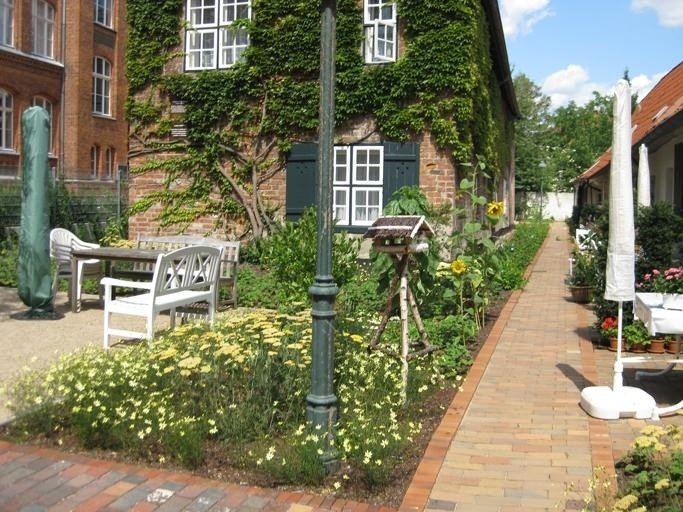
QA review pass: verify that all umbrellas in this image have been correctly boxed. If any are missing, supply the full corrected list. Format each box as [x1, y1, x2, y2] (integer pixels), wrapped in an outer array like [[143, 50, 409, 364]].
[[604, 78, 636, 395], [638, 144, 651, 205]]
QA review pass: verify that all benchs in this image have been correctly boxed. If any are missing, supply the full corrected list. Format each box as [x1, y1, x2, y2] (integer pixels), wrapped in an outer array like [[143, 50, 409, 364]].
[[97, 242, 220, 352], [107, 230, 241, 319]]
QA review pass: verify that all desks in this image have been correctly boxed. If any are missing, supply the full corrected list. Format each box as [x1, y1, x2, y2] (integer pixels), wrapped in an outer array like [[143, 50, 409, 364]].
[[67, 242, 212, 314], [626, 290, 682, 423]]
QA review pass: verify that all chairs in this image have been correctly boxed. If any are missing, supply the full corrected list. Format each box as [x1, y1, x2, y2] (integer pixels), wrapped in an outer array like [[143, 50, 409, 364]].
[[155, 234, 223, 322], [46, 224, 105, 315]]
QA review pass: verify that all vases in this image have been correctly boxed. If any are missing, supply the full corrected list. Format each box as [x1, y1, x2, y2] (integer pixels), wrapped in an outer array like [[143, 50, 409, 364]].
[[557, 198, 682, 356]]
